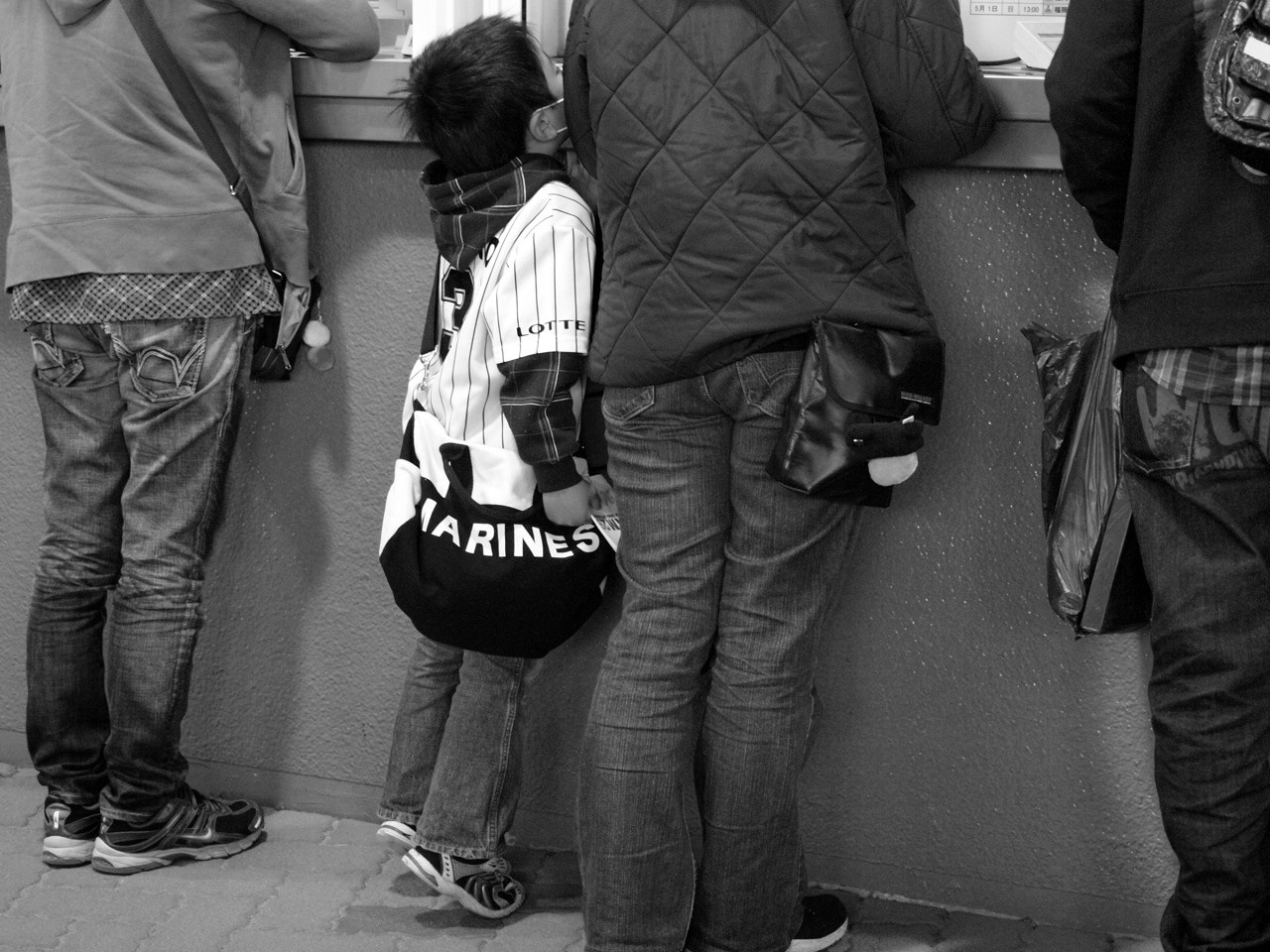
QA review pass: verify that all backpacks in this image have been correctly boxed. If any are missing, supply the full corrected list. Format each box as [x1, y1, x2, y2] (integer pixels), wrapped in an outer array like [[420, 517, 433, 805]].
[[1204, 0, 1270, 153]]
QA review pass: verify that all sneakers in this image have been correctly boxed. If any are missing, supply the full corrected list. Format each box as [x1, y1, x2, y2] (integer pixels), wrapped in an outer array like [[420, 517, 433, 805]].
[[375, 820, 419, 854], [43, 795, 102, 866], [400, 843, 527, 919], [788, 895, 848, 951], [90, 786, 265, 875]]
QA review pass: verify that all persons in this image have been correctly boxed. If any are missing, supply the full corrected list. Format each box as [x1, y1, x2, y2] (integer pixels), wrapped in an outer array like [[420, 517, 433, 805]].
[[562, 0, 994, 952], [1045, 0, 1270, 951], [381, 15, 597, 918], [1, 0, 377, 873]]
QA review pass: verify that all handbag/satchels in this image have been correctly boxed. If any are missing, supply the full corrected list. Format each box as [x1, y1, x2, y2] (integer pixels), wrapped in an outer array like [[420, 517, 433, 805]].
[[250, 267, 328, 381], [765, 322, 947, 510], [1022, 316, 1148, 636], [380, 408, 617, 654]]
